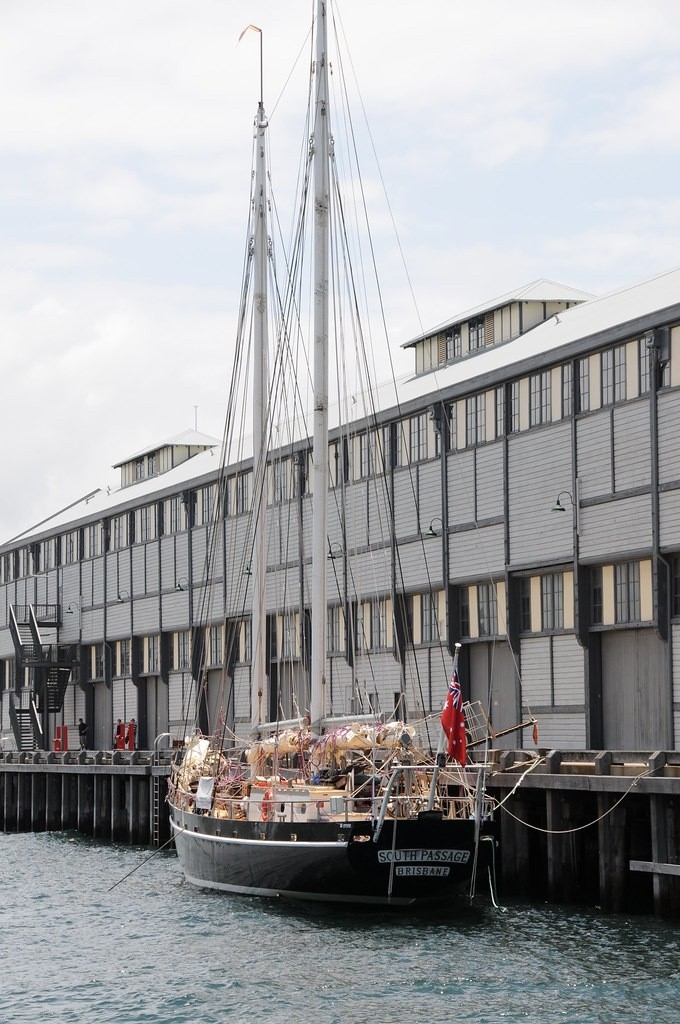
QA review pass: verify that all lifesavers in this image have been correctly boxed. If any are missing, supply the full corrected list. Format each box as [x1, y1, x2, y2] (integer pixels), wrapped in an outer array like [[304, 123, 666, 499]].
[[260, 788, 274, 821]]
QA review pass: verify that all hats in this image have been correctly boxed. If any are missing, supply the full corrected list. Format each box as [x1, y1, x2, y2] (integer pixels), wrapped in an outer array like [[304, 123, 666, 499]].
[[131, 719, 135, 721]]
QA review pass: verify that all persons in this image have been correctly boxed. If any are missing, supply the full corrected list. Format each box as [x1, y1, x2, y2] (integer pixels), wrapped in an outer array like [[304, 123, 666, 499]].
[[114, 718, 137, 750], [78, 718, 89, 750], [188, 776, 199, 793]]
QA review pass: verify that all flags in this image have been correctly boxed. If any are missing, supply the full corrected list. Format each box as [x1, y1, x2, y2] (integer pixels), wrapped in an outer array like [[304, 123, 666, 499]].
[[441, 668, 469, 768]]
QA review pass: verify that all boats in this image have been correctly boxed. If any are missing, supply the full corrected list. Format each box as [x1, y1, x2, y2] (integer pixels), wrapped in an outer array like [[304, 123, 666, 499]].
[[164, 2, 502, 914]]
